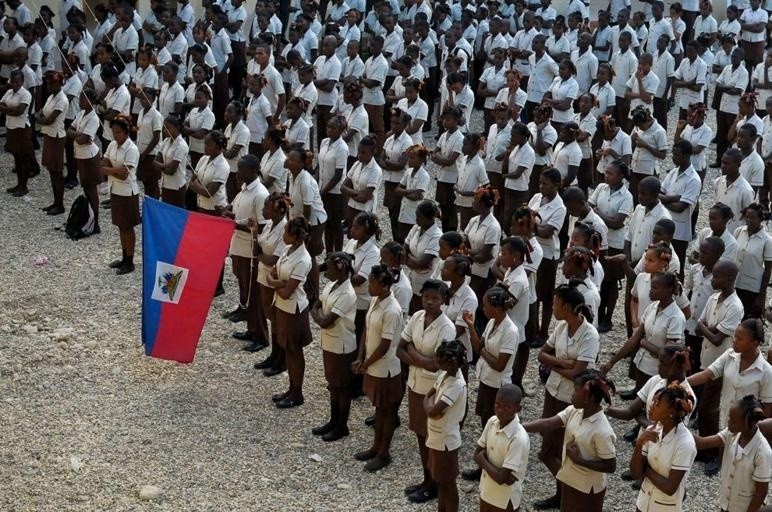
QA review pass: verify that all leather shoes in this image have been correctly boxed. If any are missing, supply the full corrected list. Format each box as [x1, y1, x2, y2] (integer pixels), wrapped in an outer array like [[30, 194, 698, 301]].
[[6, 177, 134, 275]]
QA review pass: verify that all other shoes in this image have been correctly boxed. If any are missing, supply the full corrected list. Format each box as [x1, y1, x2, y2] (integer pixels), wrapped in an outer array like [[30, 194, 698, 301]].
[[623, 423, 647, 443], [323, 426, 350, 441], [461, 467, 483, 481], [313, 421, 337, 435], [364, 415, 375, 425], [530, 333, 549, 348], [254, 356, 280, 369], [223, 307, 248, 322], [372, 415, 401, 430], [619, 387, 642, 400], [631, 479, 643, 490], [264, 366, 288, 377], [704, 455, 721, 475], [271, 391, 294, 402], [533, 495, 562, 509], [693, 448, 710, 462], [232, 332, 252, 340], [241, 343, 264, 352], [620, 470, 633, 481], [364, 456, 392, 471], [276, 394, 306, 408], [354, 448, 378, 461], [404, 485, 439, 503]]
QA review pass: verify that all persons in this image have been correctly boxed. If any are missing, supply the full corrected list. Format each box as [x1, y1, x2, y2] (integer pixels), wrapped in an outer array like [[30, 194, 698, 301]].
[[3, 1, 771, 511]]
